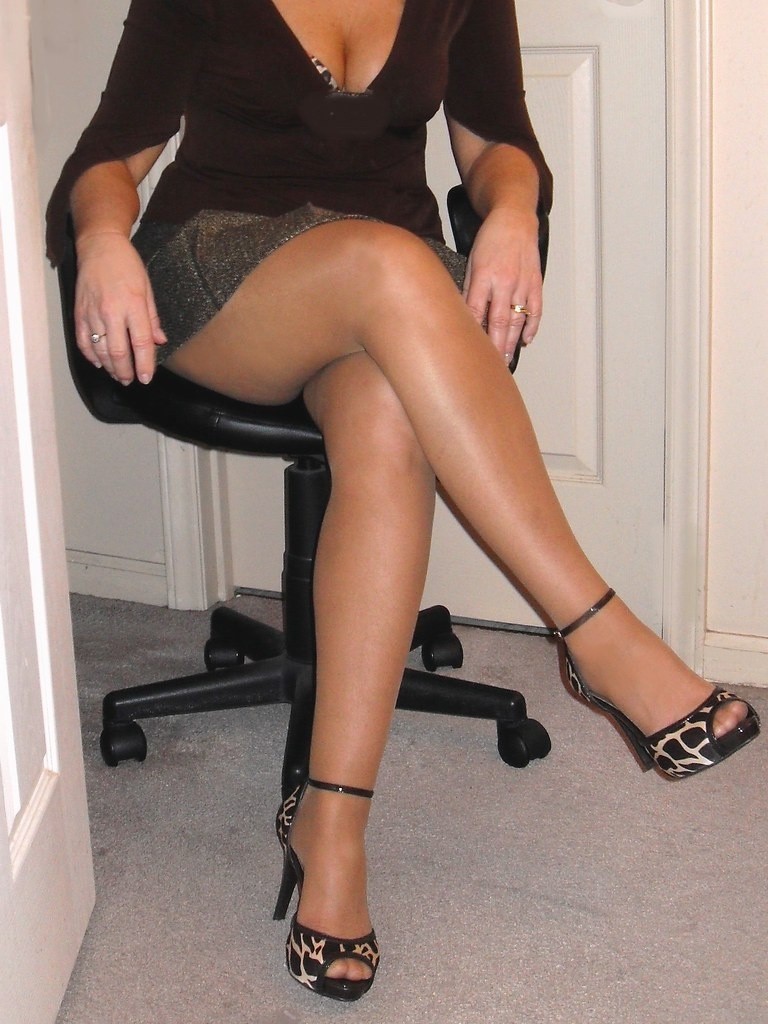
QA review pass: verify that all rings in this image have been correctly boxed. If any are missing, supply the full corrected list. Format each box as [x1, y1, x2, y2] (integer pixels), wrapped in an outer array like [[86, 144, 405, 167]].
[[511, 304, 530, 314], [89, 332, 106, 343]]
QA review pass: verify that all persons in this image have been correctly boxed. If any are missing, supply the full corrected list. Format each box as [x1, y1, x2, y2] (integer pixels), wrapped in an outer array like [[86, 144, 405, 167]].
[[45, 0, 762, 1002]]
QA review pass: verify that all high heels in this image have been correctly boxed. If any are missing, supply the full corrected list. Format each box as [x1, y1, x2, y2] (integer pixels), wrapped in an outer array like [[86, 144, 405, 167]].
[[272, 778, 381, 1002], [552, 588, 761, 781]]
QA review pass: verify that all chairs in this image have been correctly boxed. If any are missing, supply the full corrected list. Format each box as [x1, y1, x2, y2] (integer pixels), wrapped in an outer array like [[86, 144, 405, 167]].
[[55, 185, 552, 802]]
